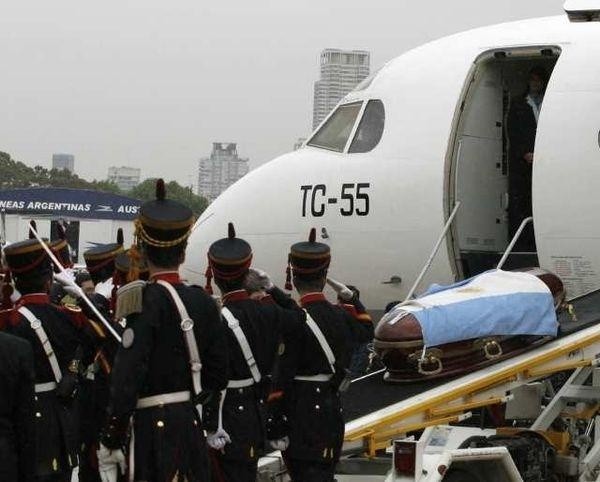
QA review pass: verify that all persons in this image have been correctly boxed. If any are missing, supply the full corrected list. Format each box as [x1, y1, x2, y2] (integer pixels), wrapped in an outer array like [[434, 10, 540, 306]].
[[505, 66, 549, 252], [2, 200, 377, 482]]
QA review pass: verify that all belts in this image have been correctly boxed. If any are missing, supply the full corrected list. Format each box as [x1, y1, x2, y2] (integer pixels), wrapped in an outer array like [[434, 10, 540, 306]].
[[226, 379, 254, 387], [136, 391, 190, 408], [35, 382, 56, 393], [295, 374, 330, 381]]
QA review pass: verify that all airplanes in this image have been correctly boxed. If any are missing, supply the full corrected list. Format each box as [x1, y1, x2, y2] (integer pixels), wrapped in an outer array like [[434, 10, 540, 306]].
[[178, 1, 599, 482]]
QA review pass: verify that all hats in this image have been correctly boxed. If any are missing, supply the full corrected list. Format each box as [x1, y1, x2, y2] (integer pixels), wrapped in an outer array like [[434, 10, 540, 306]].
[[207, 237, 252, 280], [290, 242, 330, 274], [3, 237, 148, 286], [135, 199, 192, 259]]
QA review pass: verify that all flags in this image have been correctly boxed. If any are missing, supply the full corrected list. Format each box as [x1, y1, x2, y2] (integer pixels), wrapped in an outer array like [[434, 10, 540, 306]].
[[395, 268, 559, 348]]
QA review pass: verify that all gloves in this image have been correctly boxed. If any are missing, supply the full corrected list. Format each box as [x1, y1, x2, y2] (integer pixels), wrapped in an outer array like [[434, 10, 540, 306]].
[[99, 443, 125, 482], [249, 267, 274, 290], [207, 427, 231, 449], [327, 277, 353, 300], [269, 437, 289, 450]]
